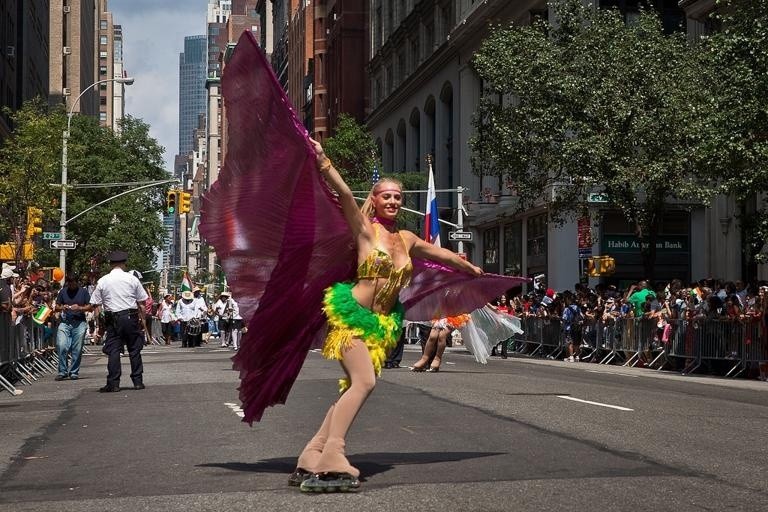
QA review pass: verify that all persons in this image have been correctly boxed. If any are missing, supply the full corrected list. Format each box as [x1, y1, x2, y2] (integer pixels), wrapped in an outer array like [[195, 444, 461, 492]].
[[135, 280, 248, 349], [89, 251, 149, 391], [413, 281, 469, 369], [483, 275, 768, 382], [0, 266, 107, 382], [293, 127, 485, 479]]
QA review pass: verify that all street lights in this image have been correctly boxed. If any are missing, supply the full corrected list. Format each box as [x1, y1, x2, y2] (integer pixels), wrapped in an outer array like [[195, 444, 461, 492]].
[[205, 262, 228, 295], [58, 77, 134, 290]]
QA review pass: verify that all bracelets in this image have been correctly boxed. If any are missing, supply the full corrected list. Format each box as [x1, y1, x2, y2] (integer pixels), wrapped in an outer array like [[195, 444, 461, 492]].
[[320, 157, 332, 171]]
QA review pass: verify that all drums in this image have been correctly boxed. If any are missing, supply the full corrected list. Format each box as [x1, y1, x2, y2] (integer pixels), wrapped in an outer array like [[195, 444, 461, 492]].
[[187, 319, 202, 336]]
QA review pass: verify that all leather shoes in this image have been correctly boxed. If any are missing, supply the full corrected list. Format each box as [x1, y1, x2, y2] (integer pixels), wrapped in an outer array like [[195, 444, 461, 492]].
[[134, 384, 144, 389], [100, 385, 119, 392], [56, 375, 78, 381]]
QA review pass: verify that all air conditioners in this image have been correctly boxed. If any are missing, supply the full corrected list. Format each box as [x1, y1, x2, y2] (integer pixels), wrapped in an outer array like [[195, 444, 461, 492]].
[[64, 6, 71, 14], [63, 47, 73, 56], [63, 88, 71, 97], [7, 46, 16, 57]]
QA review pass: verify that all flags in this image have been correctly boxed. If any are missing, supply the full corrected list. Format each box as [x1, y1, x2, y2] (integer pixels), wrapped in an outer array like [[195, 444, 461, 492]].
[[425, 164, 442, 250]]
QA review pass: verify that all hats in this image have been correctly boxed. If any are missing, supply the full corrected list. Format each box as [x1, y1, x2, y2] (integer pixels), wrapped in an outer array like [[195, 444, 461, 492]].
[[1, 262, 19, 279], [111, 252, 127, 262], [181, 287, 200, 300]]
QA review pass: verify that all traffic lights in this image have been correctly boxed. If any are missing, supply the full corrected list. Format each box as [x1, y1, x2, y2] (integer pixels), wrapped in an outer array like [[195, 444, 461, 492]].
[[583, 257, 594, 277], [177, 191, 191, 215], [166, 190, 175, 215], [26, 205, 43, 239]]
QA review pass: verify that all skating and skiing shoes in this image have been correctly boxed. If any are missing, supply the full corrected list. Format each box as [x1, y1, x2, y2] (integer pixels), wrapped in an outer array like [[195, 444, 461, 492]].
[[412, 357, 441, 372], [288, 438, 360, 491]]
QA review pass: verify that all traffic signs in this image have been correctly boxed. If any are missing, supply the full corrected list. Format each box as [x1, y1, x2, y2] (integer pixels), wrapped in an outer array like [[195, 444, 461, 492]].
[[50, 240, 76, 249], [448, 232, 472, 241]]
